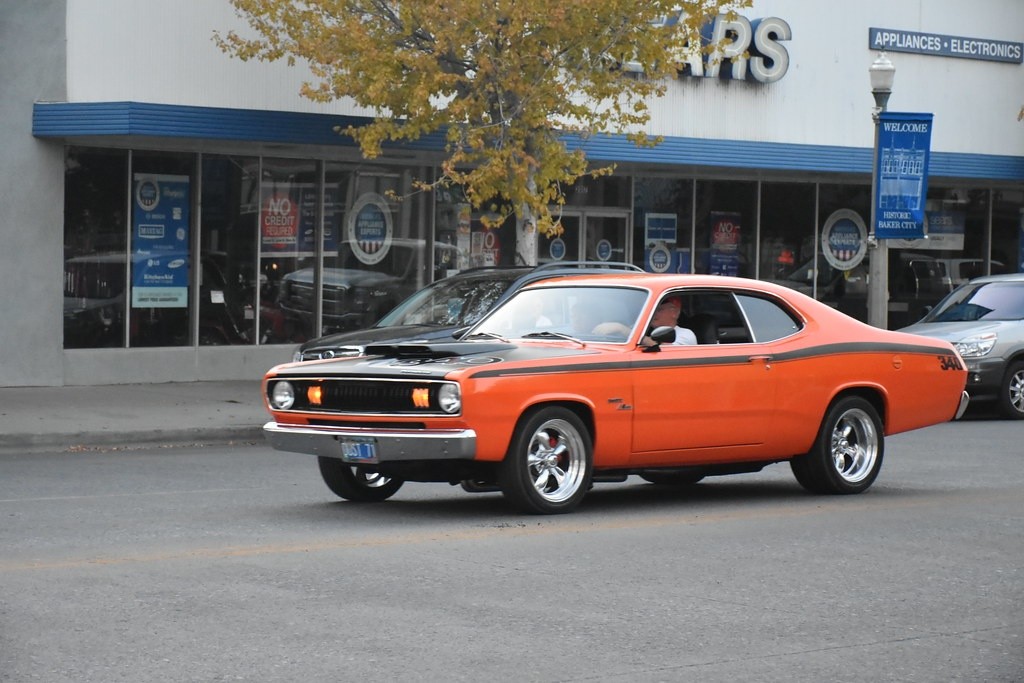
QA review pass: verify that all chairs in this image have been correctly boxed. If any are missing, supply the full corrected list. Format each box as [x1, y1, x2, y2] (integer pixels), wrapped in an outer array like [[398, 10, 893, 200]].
[[690, 312, 719, 344]]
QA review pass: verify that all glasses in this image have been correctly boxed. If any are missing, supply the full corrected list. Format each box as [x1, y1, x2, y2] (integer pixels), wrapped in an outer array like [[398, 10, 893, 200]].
[[662, 298, 669, 304]]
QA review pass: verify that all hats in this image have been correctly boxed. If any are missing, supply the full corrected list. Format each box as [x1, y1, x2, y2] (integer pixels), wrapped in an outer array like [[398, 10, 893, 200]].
[[661, 296, 683, 308]]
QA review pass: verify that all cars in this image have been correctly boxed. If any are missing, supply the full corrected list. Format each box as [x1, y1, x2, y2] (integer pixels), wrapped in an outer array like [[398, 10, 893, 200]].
[[774, 253, 946, 330], [262, 272, 971, 516], [157, 251, 303, 345], [895, 272, 1024, 421]]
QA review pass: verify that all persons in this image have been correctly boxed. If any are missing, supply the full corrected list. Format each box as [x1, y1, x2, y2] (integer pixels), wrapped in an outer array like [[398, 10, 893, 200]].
[[592, 296, 699, 348]]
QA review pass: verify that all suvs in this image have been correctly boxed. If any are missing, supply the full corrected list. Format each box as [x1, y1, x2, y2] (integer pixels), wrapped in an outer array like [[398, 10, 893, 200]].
[[290, 263, 649, 363], [275, 237, 478, 337]]
